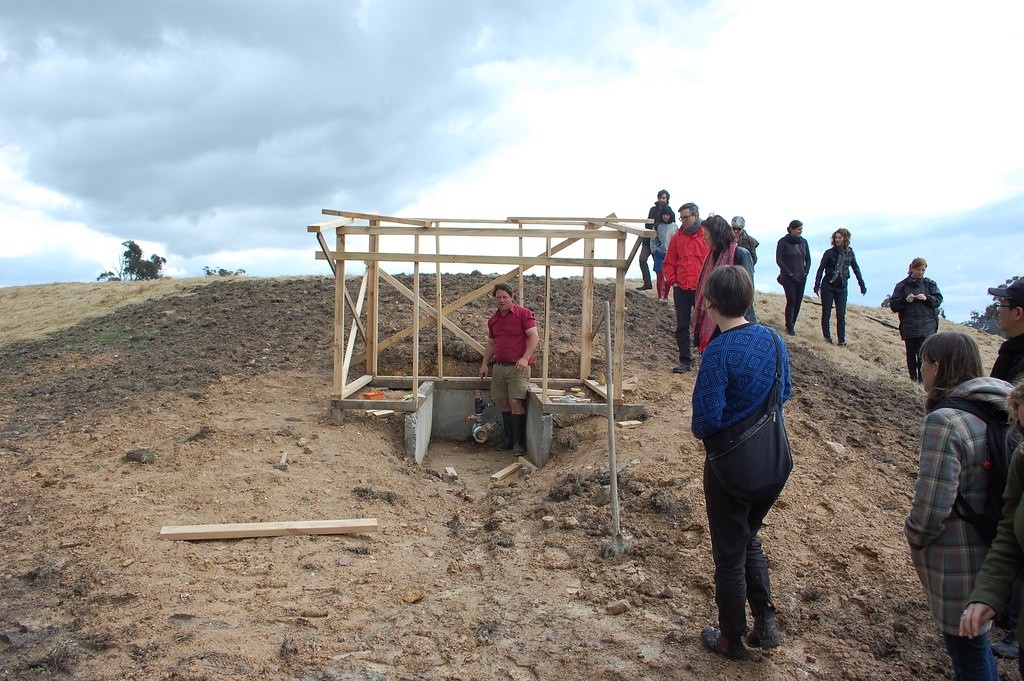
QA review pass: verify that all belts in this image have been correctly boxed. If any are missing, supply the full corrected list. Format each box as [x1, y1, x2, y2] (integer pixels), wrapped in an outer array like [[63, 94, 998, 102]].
[[495, 362, 517, 366]]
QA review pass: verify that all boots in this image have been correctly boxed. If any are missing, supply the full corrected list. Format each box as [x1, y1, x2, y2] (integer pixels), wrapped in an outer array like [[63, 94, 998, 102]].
[[496, 411, 513, 450], [511, 414, 526, 456], [700, 624, 749, 660], [746, 612, 781, 648]]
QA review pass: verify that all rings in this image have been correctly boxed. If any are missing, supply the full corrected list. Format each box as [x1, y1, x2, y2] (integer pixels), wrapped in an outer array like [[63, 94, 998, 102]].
[[962, 610, 967, 615]]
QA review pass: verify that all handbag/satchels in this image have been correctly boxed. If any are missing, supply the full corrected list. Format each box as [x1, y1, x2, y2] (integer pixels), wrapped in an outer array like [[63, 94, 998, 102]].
[[703, 381, 793, 493]]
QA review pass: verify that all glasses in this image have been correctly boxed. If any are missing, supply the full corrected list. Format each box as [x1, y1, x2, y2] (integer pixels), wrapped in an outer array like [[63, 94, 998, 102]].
[[732, 227, 742, 231], [994, 302, 1010, 313], [678, 215, 690, 222]]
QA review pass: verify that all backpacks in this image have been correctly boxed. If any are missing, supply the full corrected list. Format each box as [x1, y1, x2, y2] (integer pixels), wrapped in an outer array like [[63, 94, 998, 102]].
[[926, 395, 1010, 549]]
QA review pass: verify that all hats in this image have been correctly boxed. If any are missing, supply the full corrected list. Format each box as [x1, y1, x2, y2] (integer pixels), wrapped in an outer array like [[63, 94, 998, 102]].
[[988, 278, 1024, 298]]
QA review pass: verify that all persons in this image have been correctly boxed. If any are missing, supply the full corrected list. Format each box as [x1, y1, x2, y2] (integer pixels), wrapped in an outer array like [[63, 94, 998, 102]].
[[890, 258, 943, 383], [814, 228, 866, 347], [776, 220, 810, 335], [958, 379, 1024, 681], [987, 278, 1024, 383], [479, 282, 538, 455], [635, 189, 758, 373], [904, 331, 1015, 681], [691, 264, 794, 663]]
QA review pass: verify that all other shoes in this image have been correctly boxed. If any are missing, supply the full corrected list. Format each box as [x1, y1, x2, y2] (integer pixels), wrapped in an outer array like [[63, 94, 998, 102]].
[[636, 283, 652, 291], [787, 327, 796, 336], [990, 631, 1019, 659], [672, 362, 694, 373], [837, 340, 847, 347], [823, 337, 834, 345]]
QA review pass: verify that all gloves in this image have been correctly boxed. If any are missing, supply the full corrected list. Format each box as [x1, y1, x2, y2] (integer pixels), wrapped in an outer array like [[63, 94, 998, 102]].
[[860, 287, 867, 295], [814, 287, 820, 297]]
